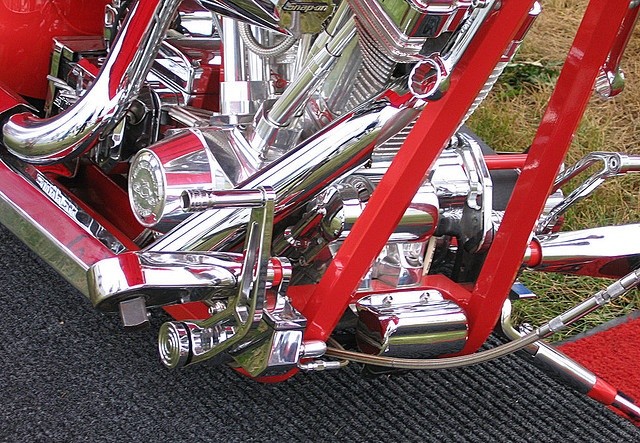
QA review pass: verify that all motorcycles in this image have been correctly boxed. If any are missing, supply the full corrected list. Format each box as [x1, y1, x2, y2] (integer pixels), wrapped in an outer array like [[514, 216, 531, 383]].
[[0, 0, 640, 429]]
